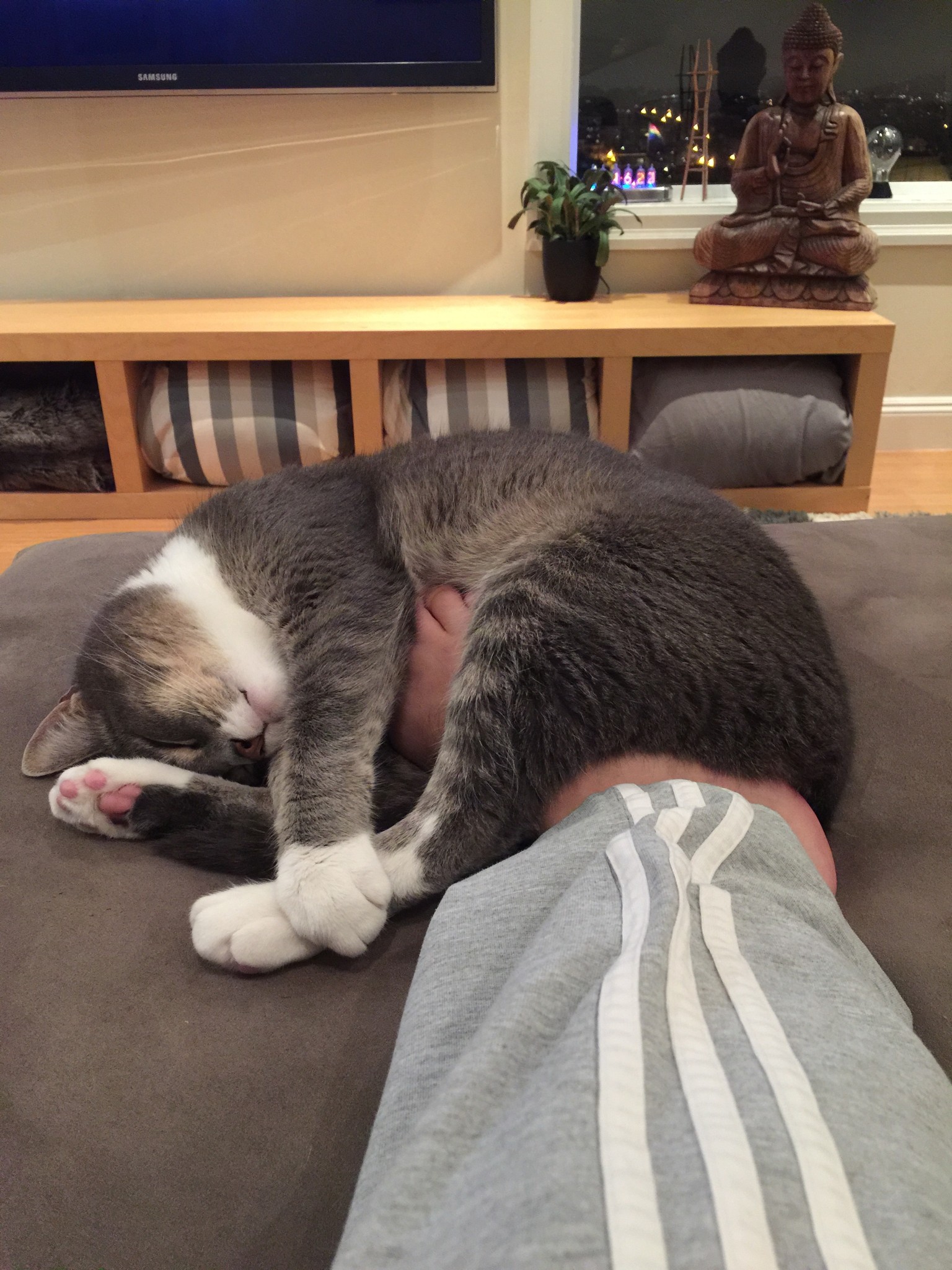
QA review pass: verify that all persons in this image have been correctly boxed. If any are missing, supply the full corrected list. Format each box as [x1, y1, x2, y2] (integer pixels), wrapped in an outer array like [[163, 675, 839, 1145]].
[[690, 3, 880, 311], [328, 577, 952, 1270], [694, 25, 767, 180]]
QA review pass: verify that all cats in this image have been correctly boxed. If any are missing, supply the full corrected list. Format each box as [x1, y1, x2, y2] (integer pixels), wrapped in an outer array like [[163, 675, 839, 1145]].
[[17, 425, 849, 975]]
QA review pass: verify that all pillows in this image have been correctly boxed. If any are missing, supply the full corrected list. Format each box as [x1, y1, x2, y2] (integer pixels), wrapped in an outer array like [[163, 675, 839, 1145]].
[[136, 358, 352, 487], [382, 358, 598, 446], [629, 356, 853, 489]]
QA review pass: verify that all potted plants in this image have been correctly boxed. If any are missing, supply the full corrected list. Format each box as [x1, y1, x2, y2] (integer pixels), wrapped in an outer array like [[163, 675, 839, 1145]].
[[507, 158, 644, 305]]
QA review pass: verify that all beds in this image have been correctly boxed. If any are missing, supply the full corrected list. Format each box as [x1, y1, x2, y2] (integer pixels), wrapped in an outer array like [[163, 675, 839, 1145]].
[[1, 508, 952, 1270]]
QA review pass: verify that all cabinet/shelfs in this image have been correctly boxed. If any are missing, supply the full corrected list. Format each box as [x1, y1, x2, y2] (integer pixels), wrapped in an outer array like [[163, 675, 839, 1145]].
[[3, 298, 895, 521]]
